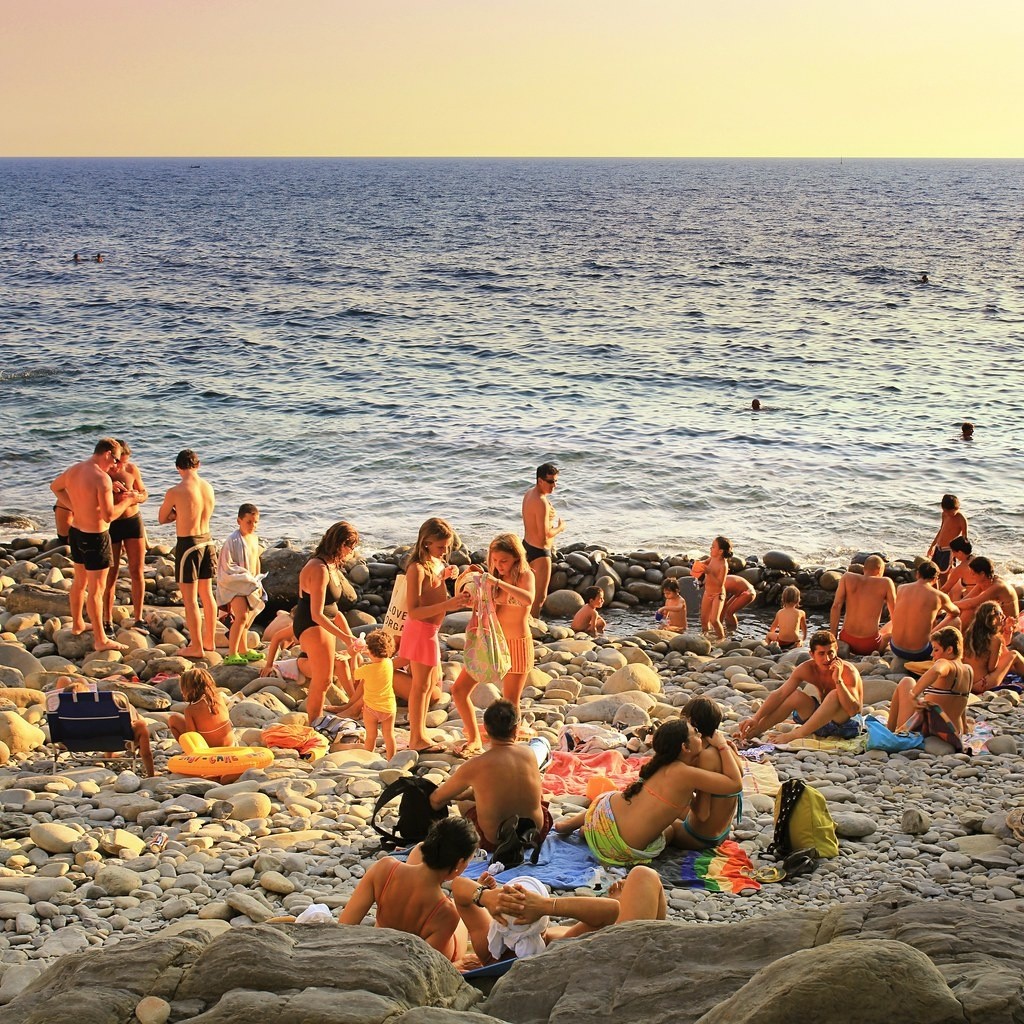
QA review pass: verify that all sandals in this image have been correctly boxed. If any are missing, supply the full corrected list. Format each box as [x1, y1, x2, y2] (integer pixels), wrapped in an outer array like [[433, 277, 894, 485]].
[[239, 649, 265, 661], [224, 654, 248, 665]]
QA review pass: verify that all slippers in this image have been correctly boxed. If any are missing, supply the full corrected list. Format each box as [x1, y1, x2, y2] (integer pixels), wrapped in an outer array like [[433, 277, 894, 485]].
[[733, 739, 753, 750], [757, 867, 787, 883], [783, 847, 819, 870], [739, 866, 758, 879], [417, 744, 447, 755], [785, 856, 819, 880], [151, 673, 180, 683], [453, 745, 482, 760], [120, 676, 140, 682]]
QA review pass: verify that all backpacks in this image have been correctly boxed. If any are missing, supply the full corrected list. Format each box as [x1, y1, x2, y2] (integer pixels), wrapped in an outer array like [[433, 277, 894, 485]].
[[312, 715, 366, 744], [759, 779, 840, 857], [372, 776, 451, 847]]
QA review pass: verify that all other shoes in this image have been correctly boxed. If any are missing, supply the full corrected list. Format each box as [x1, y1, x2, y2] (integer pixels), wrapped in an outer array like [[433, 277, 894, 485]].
[[627, 738, 642, 751]]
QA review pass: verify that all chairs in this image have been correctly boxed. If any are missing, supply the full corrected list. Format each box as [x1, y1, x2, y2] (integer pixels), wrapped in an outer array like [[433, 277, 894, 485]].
[[44, 689, 142, 775]]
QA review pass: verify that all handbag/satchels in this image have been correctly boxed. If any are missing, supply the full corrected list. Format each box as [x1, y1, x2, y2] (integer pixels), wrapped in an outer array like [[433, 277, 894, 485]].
[[382, 576, 408, 650], [462, 575, 512, 683], [865, 716, 925, 751]]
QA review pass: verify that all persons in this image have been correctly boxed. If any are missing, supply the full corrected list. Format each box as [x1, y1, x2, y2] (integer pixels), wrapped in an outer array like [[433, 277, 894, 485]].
[[673, 695, 745, 852], [752, 399, 760, 410], [523, 463, 807, 650], [831, 494, 1024, 692], [887, 626, 974, 736], [50, 438, 270, 664], [922, 274, 928, 282], [740, 631, 863, 744], [962, 423, 974, 436], [73, 253, 103, 262], [430, 698, 554, 853], [263, 518, 536, 758], [557, 720, 744, 868], [168, 668, 235, 747], [452, 866, 667, 966], [339, 816, 497, 964], [55, 676, 154, 777]]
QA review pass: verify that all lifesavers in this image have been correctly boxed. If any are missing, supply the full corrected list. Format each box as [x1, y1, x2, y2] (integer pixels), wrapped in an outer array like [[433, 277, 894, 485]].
[[461, 725, 538, 743], [168, 731, 275, 775]]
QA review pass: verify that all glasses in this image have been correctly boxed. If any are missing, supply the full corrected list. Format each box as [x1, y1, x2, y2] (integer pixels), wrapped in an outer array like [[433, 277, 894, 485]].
[[543, 478, 557, 484], [112, 454, 120, 463]]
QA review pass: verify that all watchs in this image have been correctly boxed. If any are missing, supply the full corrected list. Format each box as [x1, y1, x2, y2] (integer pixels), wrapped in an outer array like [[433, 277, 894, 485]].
[[472, 885, 491, 907]]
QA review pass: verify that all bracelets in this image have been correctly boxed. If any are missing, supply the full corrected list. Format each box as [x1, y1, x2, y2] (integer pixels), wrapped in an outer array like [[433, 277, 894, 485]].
[[909, 689, 917, 697], [552, 898, 557, 914], [720, 747, 729, 751], [835, 680, 842, 684], [495, 579, 499, 589]]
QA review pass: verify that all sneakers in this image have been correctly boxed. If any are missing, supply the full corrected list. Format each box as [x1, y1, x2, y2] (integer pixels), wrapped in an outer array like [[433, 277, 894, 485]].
[[103, 621, 114, 637], [131, 621, 150, 635]]
